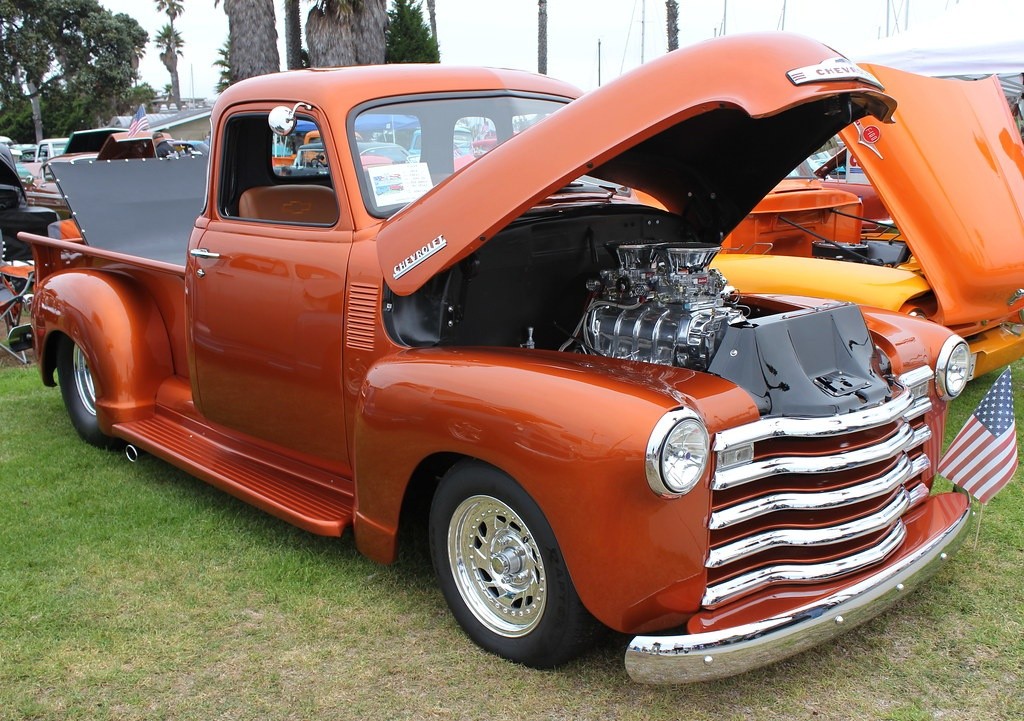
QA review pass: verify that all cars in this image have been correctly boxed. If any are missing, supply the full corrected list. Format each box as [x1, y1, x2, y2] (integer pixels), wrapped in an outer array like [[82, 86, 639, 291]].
[[0, 125, 477, 277]]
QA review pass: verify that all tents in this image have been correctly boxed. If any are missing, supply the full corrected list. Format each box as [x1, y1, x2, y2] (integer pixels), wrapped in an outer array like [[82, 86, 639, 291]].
[[281, 114, 421, 161]]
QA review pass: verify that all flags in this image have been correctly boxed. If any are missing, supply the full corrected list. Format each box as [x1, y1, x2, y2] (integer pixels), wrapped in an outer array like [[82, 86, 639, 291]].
[[937, 369, 1019, 504], [128, 104, 149, 139]]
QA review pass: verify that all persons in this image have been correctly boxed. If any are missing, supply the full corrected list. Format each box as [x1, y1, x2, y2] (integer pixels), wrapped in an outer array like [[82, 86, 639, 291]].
[[151, 133, 180, 159]]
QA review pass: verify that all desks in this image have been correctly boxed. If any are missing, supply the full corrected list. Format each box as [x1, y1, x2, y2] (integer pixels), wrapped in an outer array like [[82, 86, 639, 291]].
[[0, 266, 35, 339]]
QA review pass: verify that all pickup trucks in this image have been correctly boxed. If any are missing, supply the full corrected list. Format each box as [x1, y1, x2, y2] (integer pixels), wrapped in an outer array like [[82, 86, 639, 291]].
[[15, 30, 976, 689]]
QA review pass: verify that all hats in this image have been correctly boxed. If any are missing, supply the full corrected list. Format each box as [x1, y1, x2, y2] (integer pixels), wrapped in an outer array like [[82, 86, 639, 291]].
[[152, 132, 163, 139]]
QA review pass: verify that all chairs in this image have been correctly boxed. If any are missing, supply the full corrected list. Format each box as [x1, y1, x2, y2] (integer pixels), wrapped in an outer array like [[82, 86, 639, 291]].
[[0, 229, 27, 365], [240, 185, 338, 225]]
